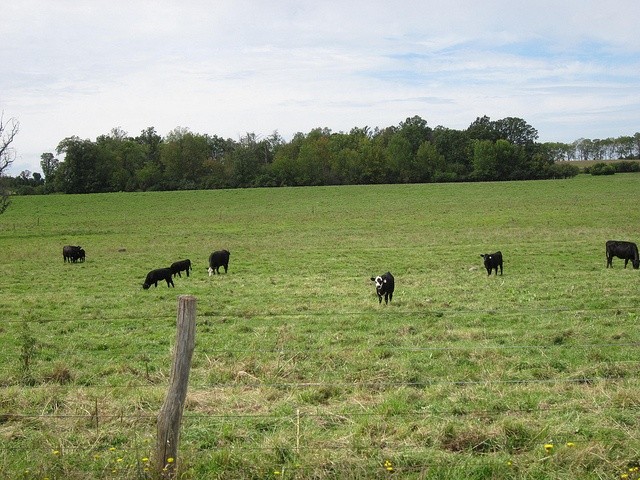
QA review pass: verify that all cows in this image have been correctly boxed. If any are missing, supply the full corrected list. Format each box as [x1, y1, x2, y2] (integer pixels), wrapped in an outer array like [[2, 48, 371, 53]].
[[205, 249, 232, 277], [605, 240, 640, 270], [479, 250, 504, 277], [171, 259, 192, 279], [139, 267, 175, 289], [370, 271, 395, 305], [63, 245, 86, 263]]
